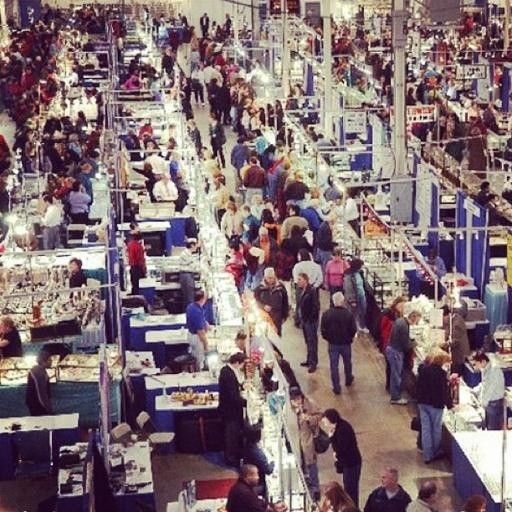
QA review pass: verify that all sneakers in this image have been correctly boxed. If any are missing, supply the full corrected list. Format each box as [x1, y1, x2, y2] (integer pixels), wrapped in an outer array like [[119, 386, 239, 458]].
[[332, 384, 342, 395], [391, 396, 413, 411], [345, 375, 354, 387], [416, 445, 447, 465]]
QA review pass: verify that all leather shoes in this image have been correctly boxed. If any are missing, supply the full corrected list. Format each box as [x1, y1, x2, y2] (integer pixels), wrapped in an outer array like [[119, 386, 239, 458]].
[[300, 361, 318, 374]]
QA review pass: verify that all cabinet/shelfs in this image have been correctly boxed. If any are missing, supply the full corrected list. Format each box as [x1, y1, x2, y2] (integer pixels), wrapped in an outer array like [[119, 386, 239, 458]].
[[207, 0, 511, 511], [2, 0, 319, 508]]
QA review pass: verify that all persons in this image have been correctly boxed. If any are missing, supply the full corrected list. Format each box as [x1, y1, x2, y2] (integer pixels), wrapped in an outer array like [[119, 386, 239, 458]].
[[1, 2, 512, 511]]
